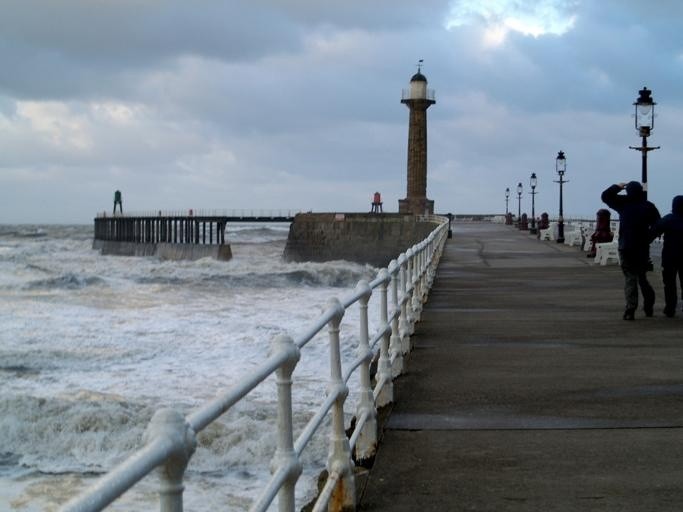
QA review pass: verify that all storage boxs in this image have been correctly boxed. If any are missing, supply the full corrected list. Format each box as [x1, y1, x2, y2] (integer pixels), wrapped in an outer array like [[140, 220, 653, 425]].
[[539, 222, 620, 266]]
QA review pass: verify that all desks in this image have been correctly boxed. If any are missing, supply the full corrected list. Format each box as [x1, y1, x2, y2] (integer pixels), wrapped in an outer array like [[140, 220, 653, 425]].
[[528, 172, 540, 234], [516, 182, 525, 222], [504, 187, 510, 214], [553, 150, 573, 241], [628, 86, 663, 198]]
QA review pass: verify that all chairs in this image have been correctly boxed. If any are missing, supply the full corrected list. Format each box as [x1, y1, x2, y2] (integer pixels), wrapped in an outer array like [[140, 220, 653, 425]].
[[645, 311, 653, 319], [663, 308, 676, 318], [622, 312, 636, 322]]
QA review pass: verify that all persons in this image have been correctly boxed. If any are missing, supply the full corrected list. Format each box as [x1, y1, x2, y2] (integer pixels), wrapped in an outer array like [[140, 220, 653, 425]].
[[602, 181, 661, 320], [649, 196, 683, 317]]
